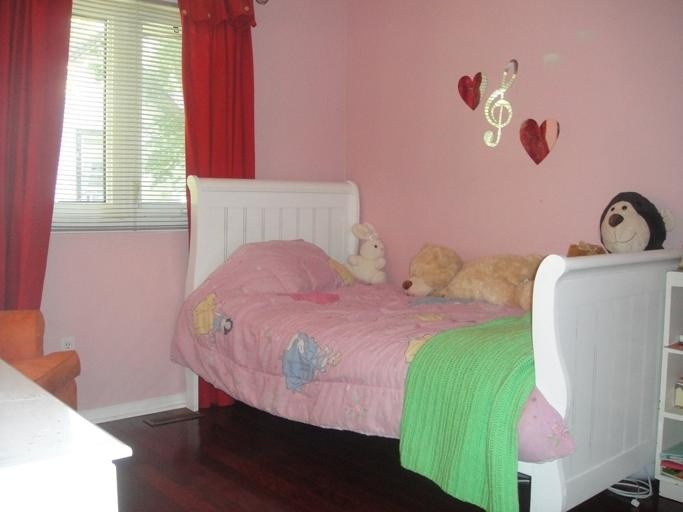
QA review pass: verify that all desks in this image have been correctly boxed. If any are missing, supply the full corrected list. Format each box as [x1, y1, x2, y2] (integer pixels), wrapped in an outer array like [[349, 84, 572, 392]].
[[0, 359, 133, 511]]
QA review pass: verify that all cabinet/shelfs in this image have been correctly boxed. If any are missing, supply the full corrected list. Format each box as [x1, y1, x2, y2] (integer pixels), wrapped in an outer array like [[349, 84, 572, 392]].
[[654, 268, 682, 504]]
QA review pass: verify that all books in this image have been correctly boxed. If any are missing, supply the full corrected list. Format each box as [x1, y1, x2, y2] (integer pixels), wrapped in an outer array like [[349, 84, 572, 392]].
[[660, 440, 683, 481]]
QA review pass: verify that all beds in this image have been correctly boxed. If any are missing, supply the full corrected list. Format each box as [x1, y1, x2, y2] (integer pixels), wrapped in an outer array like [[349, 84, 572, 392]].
[[187, 176, 682, 512]]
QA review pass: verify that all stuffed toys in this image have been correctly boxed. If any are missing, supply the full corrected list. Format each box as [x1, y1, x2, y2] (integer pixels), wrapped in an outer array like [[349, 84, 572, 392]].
[[401, 244, 545, 311], [345, 222, 387, 284], [599, 191, 666, 254]]
